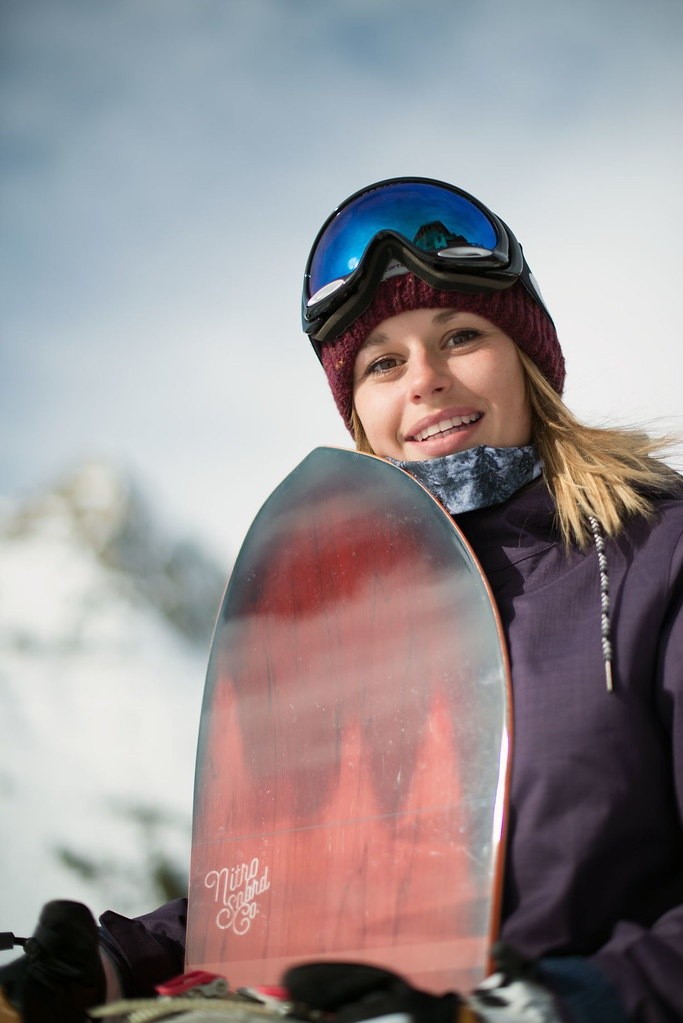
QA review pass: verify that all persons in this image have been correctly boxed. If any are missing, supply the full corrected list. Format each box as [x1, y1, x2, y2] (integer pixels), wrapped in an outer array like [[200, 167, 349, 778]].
[[1, 170, 683, 1023]]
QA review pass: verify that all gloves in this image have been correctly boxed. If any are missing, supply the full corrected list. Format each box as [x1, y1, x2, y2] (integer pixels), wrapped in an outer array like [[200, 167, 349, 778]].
[[278, 959, 488, 1022], [1, 942, 122, 1023]]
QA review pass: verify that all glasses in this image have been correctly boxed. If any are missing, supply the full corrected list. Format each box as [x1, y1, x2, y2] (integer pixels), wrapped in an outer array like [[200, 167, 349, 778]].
[[304, 175, 539, 356]]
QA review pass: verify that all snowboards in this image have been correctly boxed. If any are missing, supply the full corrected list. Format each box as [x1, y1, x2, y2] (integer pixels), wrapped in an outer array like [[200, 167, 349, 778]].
[[89, 446, 515, 1023]]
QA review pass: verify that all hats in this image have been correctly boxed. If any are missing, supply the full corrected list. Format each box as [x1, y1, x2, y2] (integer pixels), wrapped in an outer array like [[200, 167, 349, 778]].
[[306, 249, 568, 413]]
